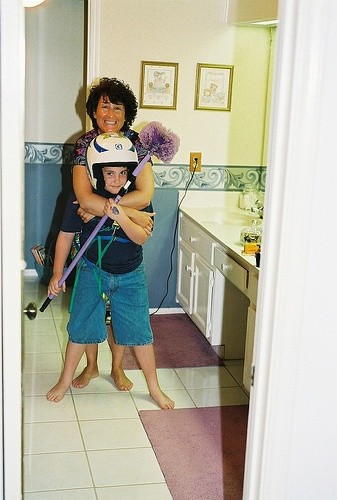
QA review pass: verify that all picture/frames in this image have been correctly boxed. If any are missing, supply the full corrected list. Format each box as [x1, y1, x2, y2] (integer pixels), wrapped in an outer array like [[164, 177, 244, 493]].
[[194, 63, 234, 111], [140, 61, 179, 110]]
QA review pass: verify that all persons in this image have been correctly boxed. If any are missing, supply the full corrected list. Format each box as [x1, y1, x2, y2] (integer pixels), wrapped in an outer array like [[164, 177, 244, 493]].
[[46, 133, 175, 411], [72, 78, 156, 392]]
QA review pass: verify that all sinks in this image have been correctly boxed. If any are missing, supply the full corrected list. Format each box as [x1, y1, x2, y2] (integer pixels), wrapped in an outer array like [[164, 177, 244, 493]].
[[209, 211, 262, 228]]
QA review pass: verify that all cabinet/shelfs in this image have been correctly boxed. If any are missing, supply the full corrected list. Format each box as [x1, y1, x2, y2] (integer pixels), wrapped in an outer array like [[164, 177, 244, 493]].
[[174, 207, 265, 395]]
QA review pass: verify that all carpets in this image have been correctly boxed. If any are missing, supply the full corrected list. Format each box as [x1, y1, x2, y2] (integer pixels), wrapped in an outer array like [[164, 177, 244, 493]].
[[138, 404, 249, 500], [107, 313, 225, 370]]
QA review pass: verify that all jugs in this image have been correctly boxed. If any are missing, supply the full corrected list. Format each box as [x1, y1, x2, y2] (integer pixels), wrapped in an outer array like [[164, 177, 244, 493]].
[[239, 184, 260, 212]]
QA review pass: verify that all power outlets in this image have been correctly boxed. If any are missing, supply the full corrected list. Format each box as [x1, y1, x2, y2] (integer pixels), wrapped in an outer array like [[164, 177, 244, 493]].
[[190, 152, 202, 172]]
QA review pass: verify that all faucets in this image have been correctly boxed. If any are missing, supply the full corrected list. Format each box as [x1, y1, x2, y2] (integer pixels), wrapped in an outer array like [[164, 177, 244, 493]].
[[250, 199, 264, 219]]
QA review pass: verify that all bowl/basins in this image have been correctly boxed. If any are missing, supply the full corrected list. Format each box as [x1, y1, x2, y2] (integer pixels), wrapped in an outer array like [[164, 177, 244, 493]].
[[34, 261, 76, 286]]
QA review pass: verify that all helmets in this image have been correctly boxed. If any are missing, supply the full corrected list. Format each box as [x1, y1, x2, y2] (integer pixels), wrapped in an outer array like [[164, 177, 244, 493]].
[[85, 132, 139, 192]]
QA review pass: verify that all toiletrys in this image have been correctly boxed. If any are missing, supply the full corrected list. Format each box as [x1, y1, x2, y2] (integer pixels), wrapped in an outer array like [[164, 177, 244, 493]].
[[239, 220, 263, 268]]
